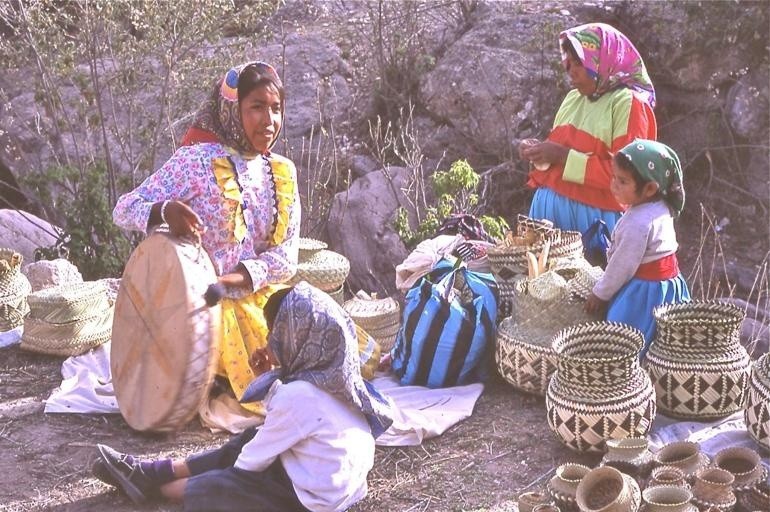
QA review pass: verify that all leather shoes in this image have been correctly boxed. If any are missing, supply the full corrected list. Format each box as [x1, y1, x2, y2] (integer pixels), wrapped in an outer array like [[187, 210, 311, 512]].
[[92, 444, 152, 505]]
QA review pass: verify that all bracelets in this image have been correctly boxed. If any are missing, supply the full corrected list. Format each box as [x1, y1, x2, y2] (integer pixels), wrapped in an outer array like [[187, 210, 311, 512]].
[[160, 200, 170, 224]]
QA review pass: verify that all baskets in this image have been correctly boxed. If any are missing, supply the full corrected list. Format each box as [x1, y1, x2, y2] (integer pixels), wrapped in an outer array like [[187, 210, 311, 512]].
[[342, 297, 401, 352], [23, 280, 115, 357]]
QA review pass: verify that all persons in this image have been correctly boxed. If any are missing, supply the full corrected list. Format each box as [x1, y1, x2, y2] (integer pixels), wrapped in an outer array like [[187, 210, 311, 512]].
[[583, 139, 691, 365], [518, 23, 657, 248], [91, 280, 395, 512], [112, 61, 381, 417]]
